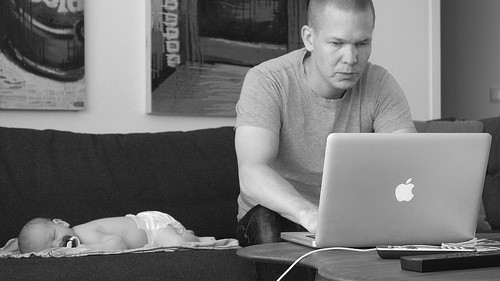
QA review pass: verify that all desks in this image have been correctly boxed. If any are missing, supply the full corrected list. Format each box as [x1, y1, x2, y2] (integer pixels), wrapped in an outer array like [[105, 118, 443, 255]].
[[236, 234, 500, 280]]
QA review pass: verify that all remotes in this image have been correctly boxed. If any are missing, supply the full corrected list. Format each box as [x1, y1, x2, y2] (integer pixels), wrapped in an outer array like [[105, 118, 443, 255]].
[[400, 251, 500, 273], [376, 243, 478, 260]]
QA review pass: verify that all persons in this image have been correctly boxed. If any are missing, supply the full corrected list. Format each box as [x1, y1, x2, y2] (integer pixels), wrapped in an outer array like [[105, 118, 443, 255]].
[[18, 211, 200, 255], [234, 0, 420, 280]]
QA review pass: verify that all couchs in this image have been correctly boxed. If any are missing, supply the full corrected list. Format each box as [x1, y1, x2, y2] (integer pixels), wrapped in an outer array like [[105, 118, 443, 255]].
[[0, 117, 500, 281]]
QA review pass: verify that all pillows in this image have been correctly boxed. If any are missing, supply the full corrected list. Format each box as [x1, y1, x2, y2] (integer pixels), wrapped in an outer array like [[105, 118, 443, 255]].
[[412, 120, 493, 234]]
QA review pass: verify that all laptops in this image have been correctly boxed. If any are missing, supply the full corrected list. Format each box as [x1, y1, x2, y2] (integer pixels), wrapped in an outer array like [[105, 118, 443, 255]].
[[280, 132, 493, 250]]
